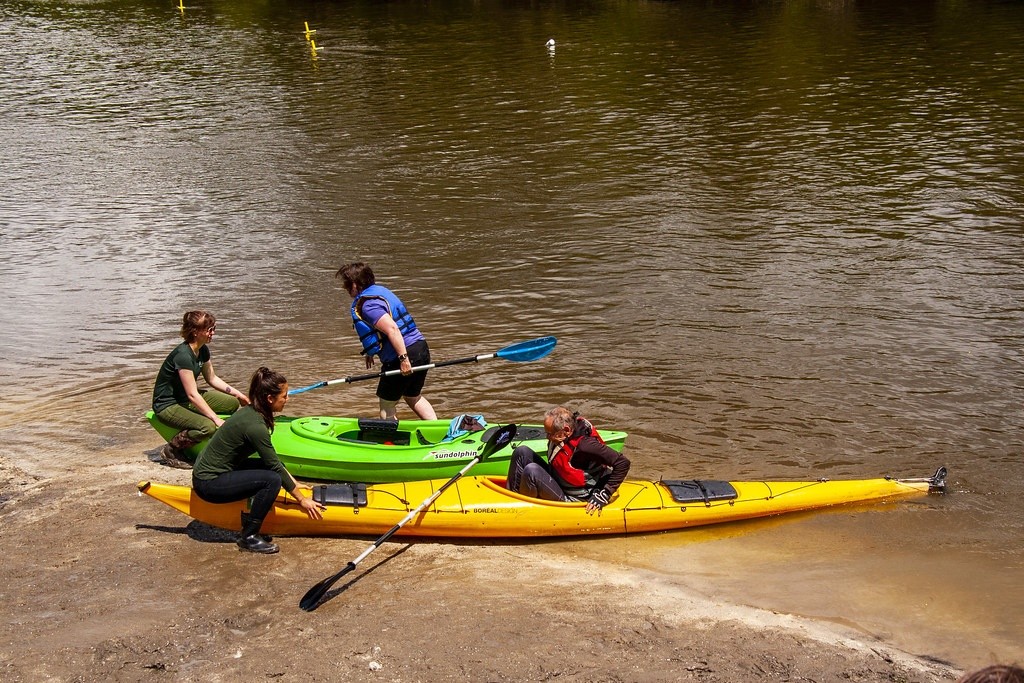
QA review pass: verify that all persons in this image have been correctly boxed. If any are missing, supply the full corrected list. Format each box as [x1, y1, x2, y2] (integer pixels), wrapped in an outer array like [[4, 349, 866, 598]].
[[153, 309, 252, 467], [342, 261, 438, 423], [507, 405, 632, 516], [191, 365, 327, 553]]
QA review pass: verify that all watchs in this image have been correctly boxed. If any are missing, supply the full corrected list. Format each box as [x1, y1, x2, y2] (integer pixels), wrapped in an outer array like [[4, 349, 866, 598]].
[[397, 354, 408, 362]]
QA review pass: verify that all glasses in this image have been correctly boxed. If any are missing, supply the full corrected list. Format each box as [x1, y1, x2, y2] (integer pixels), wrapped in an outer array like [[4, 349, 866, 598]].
[[203, 323, 217, 333]]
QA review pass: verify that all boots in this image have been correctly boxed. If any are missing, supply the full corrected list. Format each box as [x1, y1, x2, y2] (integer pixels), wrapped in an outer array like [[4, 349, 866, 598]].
[[160, 428, 202, 469], [236, 510, 280, 553]]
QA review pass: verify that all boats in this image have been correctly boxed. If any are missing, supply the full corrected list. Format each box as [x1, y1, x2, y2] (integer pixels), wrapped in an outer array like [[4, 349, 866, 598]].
[[136, 465, 950, 542], [145, 393, 630, 495]]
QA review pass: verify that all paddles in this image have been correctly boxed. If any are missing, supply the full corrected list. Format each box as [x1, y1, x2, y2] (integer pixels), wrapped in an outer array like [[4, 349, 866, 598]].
[[299, 422, 519, 612], [285, 336, 557, 395]]
